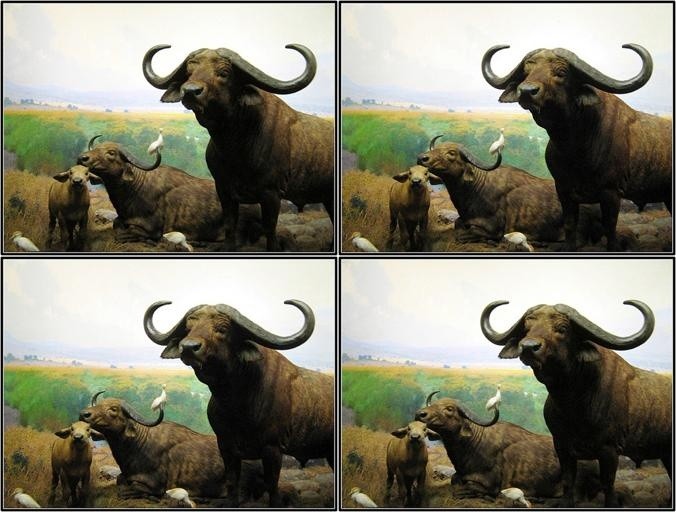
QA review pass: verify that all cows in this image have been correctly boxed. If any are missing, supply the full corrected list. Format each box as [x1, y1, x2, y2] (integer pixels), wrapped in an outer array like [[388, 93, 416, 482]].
[[414, 390, 604, 508], [45, 164, 103, 252], [385, 164, 442, 252], [142, 43, 335, 252], [480, 42, 672, 252], [78, 390, 267, 508], [480, 299, 672, 508], [416, 134, 604, 252], [143, 299, 334, 508], [77, 134, 266, 252], [45, 421, 106, 508], [382, 421, 440, 508]]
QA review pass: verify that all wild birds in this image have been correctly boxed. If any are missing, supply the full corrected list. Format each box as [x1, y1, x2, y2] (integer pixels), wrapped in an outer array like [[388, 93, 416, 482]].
[[501, 487, 532, 508], [8, 230, 41, 252], [147, 128, 164, 155], [166, 488, 197, 508], [162, 231, 194, 252], [347, 487, 378, 508], [10, 487, 41, 508], [504, 231, 535, 252], [347, 232, 379, 252], [488, 127, 513, 155], [486, 384, 502, 411], [151, 384, 167, 412]]
